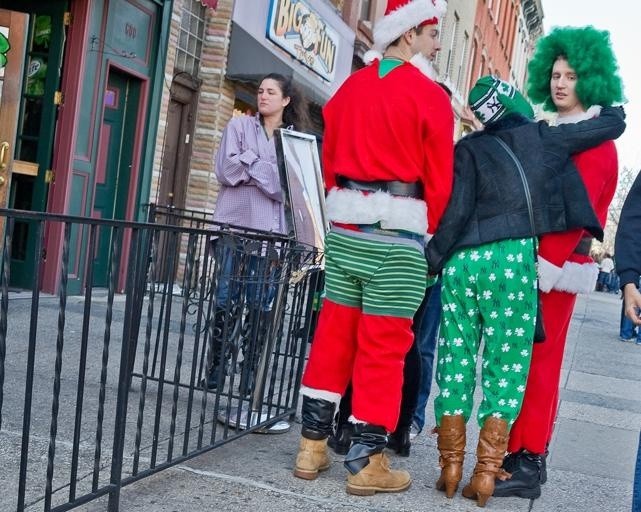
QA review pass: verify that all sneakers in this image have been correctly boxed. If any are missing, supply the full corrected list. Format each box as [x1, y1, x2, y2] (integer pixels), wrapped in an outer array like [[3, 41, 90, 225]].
[[327, 422, 419, 456], [493, 451, 547, 499], [619, 336, 641, 345]]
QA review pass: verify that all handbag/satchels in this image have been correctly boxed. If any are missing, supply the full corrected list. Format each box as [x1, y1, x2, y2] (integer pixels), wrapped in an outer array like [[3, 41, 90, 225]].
[[534, 277, 545, 342]]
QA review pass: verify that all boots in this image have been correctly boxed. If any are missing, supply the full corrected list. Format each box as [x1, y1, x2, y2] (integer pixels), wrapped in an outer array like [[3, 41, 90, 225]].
[[462, 416, 512, 508], [294, 435, 330, 481], [239, 308, 272, 395], [346, 450, 412, 495], [436, 416, 466, 498], [200, 304, 243, 389]]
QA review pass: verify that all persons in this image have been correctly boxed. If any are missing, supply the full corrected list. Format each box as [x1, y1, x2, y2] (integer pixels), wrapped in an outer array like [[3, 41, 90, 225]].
[[292, 0, 456, 497], [614, 170, 641, 326], [198, 70, 314, 399], [620, 277, 641, 345], [423, 73, 627, 507], [408, 84, 452, 441], [492, 25, 628, 500], [590, 249, 620, 295]]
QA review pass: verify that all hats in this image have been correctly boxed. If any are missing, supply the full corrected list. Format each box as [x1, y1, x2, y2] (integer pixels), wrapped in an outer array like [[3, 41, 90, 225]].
[[363, 0, 446, 66], [467, 75, 534, 128]]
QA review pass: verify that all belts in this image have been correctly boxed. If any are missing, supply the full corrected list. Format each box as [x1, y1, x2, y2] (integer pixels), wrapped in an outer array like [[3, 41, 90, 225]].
[[335, 174, 423, 197]]
